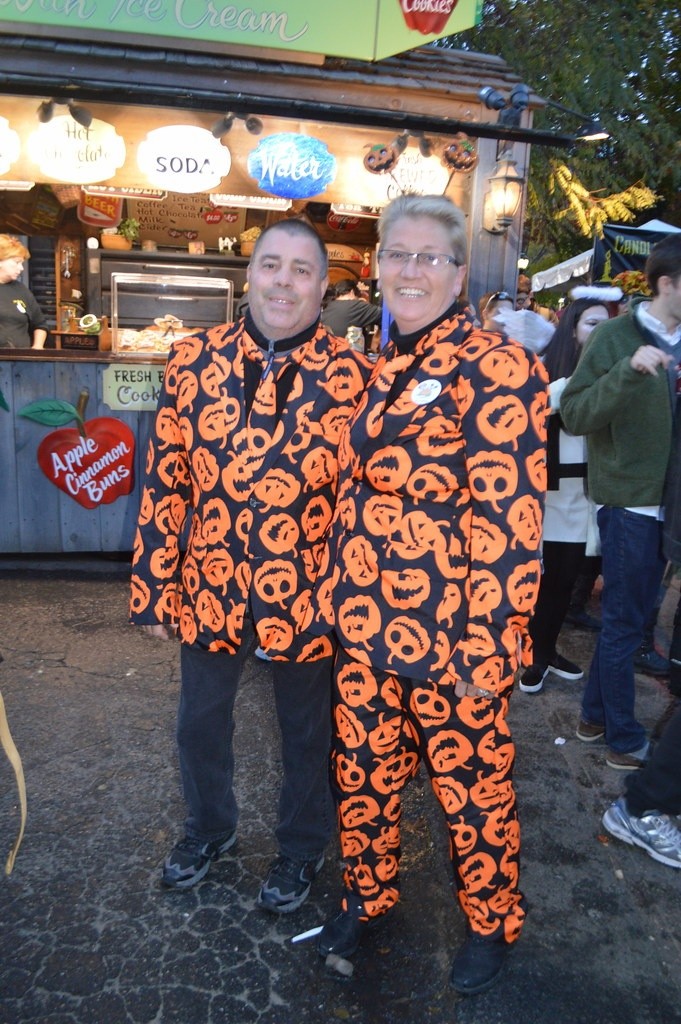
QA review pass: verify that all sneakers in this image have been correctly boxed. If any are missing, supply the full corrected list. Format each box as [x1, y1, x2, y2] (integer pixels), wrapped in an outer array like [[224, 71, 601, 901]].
[[158, 828, 239, 890], [256, 848, 326, 914], [318, 904, 379, 958], [449, 934, 507, 994], [601, 792, 681, 869]]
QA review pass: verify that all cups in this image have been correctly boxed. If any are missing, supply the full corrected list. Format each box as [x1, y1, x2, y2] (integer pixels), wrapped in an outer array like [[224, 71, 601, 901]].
[[83, 318, 104, 336]]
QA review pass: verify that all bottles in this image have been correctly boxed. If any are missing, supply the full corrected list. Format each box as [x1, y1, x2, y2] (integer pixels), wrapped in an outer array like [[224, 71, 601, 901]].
[[61, 306, 75, 333], [345, 327, 364, 354]]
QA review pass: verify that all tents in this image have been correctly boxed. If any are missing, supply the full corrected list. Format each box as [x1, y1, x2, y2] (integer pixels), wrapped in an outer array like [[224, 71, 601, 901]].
[[531, 218, 681, 292]]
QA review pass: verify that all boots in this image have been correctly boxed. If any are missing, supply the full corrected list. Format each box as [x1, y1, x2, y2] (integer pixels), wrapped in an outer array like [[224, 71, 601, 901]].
[[632, 607, 675, 676]]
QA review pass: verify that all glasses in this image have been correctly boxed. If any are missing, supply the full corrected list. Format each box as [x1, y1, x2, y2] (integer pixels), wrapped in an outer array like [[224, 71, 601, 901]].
[[376, 248, 463, 271], [486, 292, 508, 306]]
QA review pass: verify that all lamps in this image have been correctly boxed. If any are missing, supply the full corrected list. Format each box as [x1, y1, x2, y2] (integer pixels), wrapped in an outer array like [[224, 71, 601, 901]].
[[389, 123, 436, 156], [482, 140, 526, 235], [35, 95, 93, 126], [479, 80, 530, 126], [212, 110, 262, 139]]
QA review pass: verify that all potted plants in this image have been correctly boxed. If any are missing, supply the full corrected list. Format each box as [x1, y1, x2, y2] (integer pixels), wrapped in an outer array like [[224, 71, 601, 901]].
[[239, 226, 262, 255], [101, 218, 141, 250]]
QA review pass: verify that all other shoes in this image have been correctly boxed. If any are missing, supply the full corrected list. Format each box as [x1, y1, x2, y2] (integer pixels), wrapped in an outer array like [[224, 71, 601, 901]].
[[518, 661, 550, 693], [549, 654, 584, 680], [563, 611, 603, 632], [575, 714, 607, 743], [606, 738, 659, 772]]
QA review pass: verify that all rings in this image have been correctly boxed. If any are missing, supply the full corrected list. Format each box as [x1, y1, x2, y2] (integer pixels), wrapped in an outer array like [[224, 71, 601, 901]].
[[477, 689, 489, 697]]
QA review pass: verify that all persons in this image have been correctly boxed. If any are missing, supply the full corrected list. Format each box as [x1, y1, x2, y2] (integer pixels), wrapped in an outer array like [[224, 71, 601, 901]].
[[516, 274, 559, 327], [128, 221, 376, 911], [518, 232, 681, 868], [0, 233, 47, 349], [479, 290, 514, 333], [300, 191, 550, 1002], [319, 279, 382, 354]]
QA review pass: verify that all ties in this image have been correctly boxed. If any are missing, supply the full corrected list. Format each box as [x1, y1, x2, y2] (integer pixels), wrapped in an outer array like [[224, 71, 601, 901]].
[[349, 314, 460, 455], [237, 331, 312, 482]]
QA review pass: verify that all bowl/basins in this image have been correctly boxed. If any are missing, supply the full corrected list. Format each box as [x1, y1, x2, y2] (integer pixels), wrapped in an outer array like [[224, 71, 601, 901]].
[[328, 259, 364, 285], [101, 234, 132, 250], [241, 241, 255, 256]]
